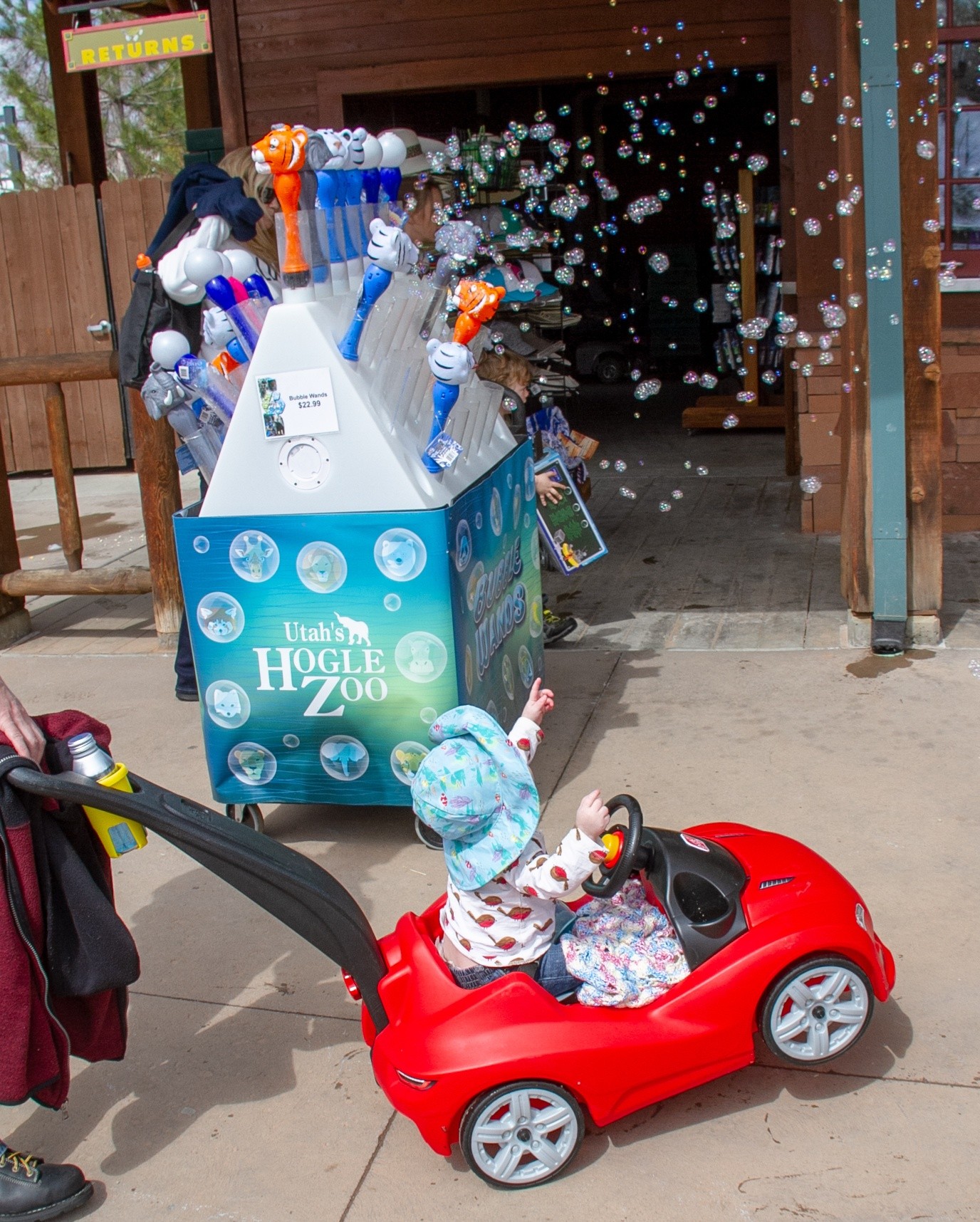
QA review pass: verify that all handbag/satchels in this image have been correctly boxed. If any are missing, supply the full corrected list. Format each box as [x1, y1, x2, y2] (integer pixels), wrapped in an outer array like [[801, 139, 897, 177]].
[[119, 260, 203, 391]]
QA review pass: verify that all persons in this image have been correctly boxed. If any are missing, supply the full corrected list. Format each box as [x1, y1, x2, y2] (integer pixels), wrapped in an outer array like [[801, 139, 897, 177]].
[[174, 131, 578, 701], [409, 678, 612, 996], [0, 679, 94, 1222]]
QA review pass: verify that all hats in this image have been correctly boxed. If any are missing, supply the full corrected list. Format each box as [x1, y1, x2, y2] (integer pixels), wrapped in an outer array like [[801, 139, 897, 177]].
[[491, 319, 537, 355], [411, 704, 539, 890], [474, 259, 562, 302], [473, 206, 559, 252], [378, 128, 433, 178]]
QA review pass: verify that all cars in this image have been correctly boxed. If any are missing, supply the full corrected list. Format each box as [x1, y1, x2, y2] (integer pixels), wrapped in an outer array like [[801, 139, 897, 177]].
[[340, 795, 897, 1193]]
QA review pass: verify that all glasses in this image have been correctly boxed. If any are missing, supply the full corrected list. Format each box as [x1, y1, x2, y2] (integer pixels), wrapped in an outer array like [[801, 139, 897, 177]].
[[257, 187, 274, 204]]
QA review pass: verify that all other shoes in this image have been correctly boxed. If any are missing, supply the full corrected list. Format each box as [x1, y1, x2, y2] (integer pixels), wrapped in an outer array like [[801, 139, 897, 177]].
[[0, 1138, 96, 1222], [540, 593, 577, 646]]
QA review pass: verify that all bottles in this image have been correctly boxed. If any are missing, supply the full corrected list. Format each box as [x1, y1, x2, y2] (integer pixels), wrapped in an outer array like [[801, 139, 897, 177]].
[[67, 732, 116, 783]]
